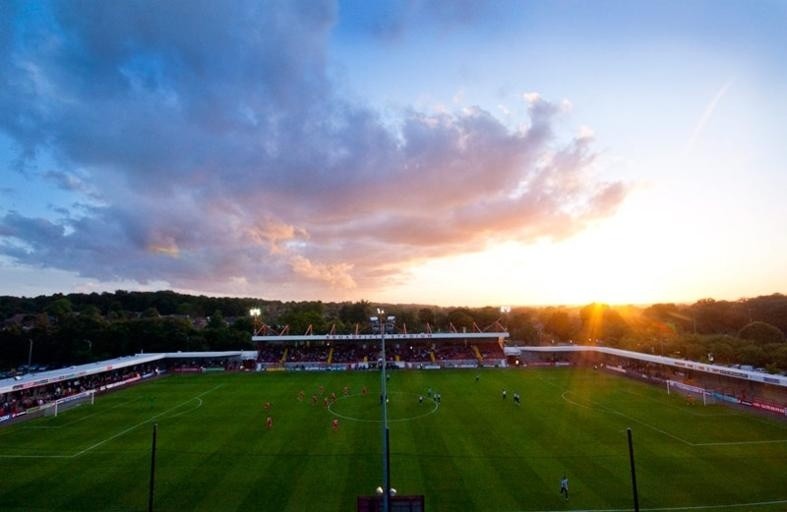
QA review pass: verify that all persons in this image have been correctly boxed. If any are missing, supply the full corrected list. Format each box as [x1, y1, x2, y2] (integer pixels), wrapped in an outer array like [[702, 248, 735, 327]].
[[560, 475, 569, 502], [501, 390, 508, 400], [165, 357, 257, 372], [331, 417, 339, 432], [256, 339, 505, 363], [0, 358, 163, 418], [600, 353, 747, 405], [475, 375, 480, 382], [508, 350, 592, 365], [262, 400, 272, 413], [296, 374, 444, 409], [514, 393, 520, 407], [264, 414, 273, 431]]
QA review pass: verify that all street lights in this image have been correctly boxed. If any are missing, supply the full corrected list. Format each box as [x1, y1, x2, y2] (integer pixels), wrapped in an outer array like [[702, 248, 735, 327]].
[[369, 308, 396, 512], [250, 308, 262, 336]]
